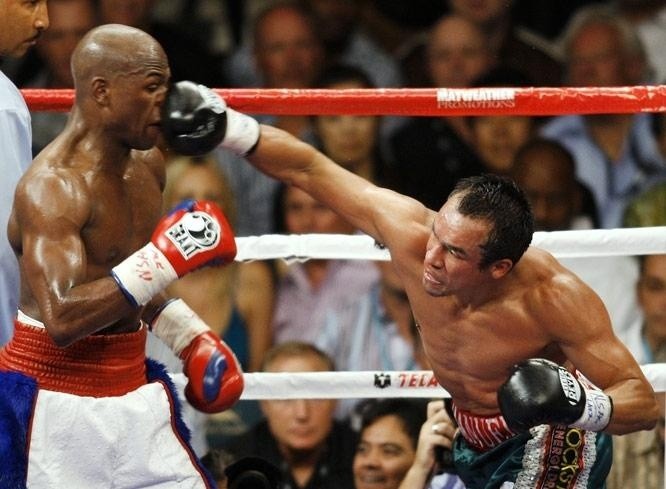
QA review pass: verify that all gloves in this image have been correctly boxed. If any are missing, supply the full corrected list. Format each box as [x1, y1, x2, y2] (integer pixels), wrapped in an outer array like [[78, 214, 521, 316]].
[[159, 79, 262, 161], [145, 295, 245, 416], [493, 354, 614, 437], [107, 196, 239, 311]]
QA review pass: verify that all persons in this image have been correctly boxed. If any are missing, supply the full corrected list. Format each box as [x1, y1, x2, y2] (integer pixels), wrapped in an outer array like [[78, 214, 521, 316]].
[[150, 75, 666, 488], [0, 17, 246, 489], [611, 338, 666, 489], [398, 398, 473, 489], [16, 1, 666, 432], [1, 0, 50, 356], [205, 335, 360, 484], [349, 396, 440, 487]]
